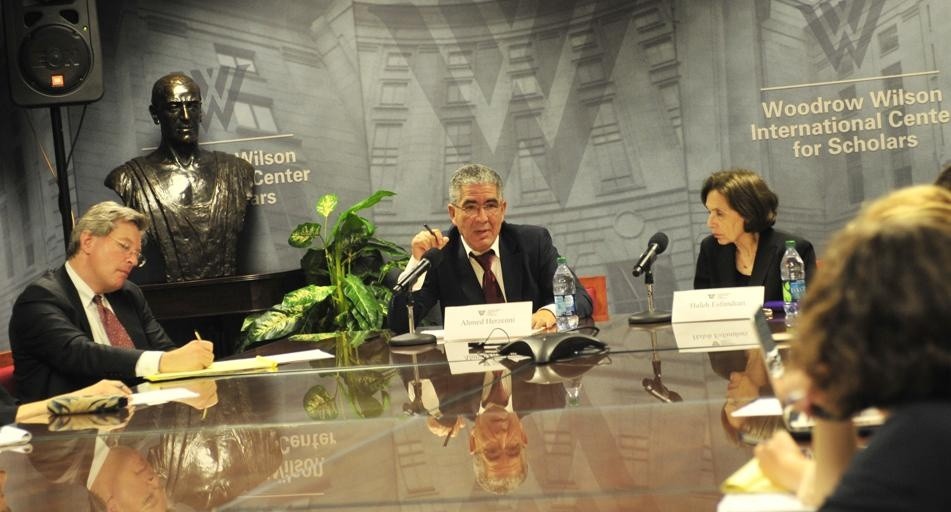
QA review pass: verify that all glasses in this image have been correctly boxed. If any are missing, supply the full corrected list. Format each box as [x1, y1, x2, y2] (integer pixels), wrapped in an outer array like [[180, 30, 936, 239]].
[[451, 202, 501, 214], [484, 446, 519, 459], [110, 234, 146, 267]]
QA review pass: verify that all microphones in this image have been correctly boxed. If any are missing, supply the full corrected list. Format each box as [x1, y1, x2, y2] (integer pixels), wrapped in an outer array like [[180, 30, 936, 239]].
[[641, 377, 684, 404], [392, 247, 443, 294], [632, 231, 669, 277]]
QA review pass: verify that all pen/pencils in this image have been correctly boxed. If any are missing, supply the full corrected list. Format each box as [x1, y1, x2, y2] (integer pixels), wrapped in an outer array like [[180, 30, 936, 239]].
[[423, 223, 437, 242], [193, 329, 202, 341], [202, 408, 207, 419], [443, 432, 452, 448]]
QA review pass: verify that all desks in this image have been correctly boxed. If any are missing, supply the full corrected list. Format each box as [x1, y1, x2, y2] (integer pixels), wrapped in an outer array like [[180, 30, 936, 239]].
[[0, 314, 874, 512]]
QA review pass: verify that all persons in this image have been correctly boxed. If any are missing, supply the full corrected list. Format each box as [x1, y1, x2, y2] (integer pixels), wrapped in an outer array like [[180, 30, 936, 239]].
[[103, 73, 254, 286], [707, 347, 785, 448], [693, 170, 817, 302], [755, 185, 951, 512], [390, 348, 569, 497], [387, 164, 593, 334], [17, 404, 175, 512], [8, 201, 214, 404]]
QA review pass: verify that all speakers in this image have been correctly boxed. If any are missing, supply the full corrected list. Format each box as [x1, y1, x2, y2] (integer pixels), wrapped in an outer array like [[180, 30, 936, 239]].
[[0, 0, 107, 108]]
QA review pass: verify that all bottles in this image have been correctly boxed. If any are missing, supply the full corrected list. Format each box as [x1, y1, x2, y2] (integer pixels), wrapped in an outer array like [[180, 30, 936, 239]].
[[553, 256, 579, 332], [780, 240, 805, 327], [562, 378, 583, 407]]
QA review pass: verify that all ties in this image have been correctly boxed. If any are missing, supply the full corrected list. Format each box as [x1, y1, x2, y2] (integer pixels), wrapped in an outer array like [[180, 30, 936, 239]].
[[469, 251, 506, 302], [482, 371, 508, 406], [93, 295, 136, 349]]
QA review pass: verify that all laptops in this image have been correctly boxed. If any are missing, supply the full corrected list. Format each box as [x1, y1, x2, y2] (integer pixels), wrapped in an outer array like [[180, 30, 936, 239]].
[[750, 302, 884, 438]]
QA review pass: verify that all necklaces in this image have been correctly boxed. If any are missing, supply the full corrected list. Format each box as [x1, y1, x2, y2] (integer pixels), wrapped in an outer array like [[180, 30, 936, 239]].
[[736, 249, 755, 269]]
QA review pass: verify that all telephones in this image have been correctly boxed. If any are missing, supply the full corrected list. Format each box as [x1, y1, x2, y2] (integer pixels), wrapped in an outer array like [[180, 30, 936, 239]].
[[497, 331, 605, 366], [498, 351, 610, 385]]
